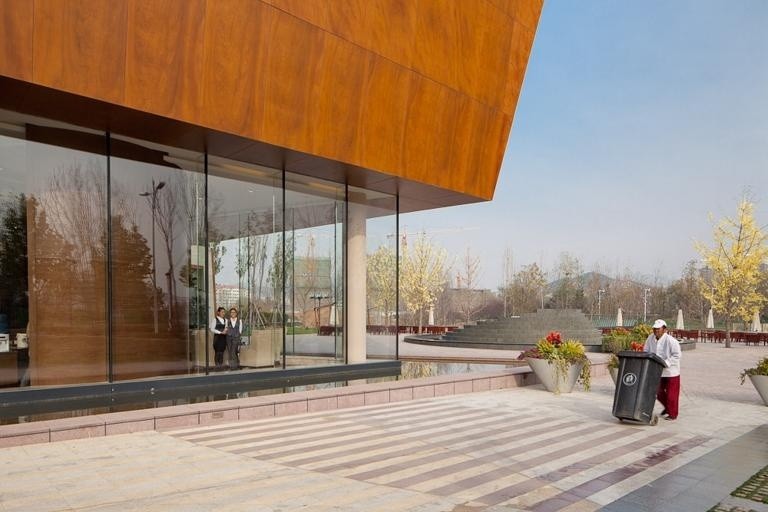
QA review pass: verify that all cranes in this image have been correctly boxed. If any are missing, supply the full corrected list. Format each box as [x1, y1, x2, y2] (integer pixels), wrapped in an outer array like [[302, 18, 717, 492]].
[[385, 222, 432, 258]]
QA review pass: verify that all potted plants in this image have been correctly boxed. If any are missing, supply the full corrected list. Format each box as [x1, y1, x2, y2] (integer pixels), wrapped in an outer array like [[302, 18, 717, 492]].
[[737, 357, 767, 406]]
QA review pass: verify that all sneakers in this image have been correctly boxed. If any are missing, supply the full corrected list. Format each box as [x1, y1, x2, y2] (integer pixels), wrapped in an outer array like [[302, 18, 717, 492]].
[[661, 410, 676, 419]]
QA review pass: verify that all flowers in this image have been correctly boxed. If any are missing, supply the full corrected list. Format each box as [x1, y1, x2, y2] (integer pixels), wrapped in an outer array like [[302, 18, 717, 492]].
[[610, 342, 644, 363], [517, 332, 597, 392]]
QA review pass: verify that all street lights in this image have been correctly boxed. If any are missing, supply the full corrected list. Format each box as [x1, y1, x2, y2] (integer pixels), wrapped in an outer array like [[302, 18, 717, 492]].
[[708, 284, 718, 310], [139, 180, 167, 336], [309, 292, 331, 336], [643, 287, 651, 322], [596, 289, 605, 319]]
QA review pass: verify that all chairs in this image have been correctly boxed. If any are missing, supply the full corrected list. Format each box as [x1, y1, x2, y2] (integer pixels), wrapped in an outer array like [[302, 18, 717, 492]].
[[595, 325, 766, 348], [319, 324, 459, 337]]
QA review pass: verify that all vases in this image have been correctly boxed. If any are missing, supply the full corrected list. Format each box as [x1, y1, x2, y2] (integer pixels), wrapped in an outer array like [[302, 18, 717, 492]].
[[608, 365, 620, 391], [525, 356, 584, 394]]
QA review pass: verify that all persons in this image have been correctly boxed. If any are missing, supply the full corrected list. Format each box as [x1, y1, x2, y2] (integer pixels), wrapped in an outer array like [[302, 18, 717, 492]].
[[643, 318, 681, 420], [226, 308, 243, 370], [210, 307, 228, 369]]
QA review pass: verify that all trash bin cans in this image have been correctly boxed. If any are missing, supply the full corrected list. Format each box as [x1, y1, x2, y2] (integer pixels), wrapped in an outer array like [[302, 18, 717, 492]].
[[612, 350, 667, 426]]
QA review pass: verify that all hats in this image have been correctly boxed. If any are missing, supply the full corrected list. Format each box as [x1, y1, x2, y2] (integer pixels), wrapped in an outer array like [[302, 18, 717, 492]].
[[652, 319, 667, 328]]
